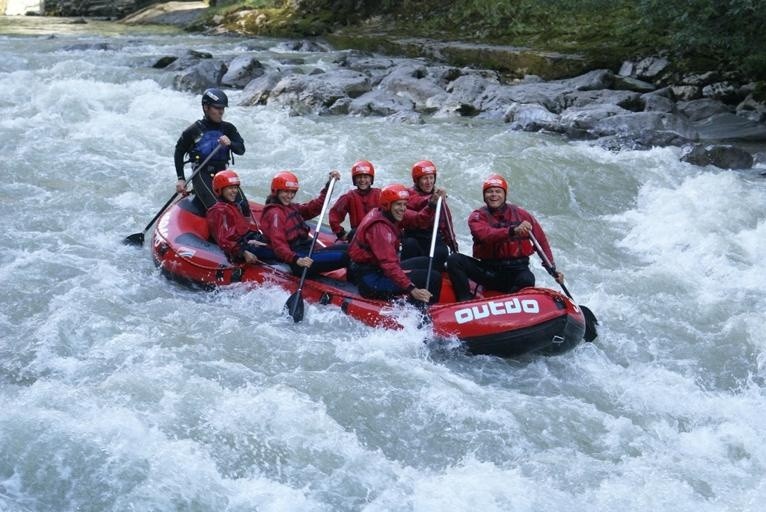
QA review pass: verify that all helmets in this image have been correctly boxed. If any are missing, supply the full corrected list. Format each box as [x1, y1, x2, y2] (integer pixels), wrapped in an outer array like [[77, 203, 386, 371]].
[[352, 161, 374, 185], [271, 171, 298, 196], [412, 160, 437, 185], [202, 88, 228, 108], [482, 176, 507, 202], [212, 170, 240, 195], [378, 184, 410, 211]]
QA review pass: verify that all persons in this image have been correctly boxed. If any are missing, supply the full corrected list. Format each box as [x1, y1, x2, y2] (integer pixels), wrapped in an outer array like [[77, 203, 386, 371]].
[[172, 88, 245, 211], [259, 172, 349, 279], [345, 184, 433, 304], [203, 169, 277, 265], [446, 174, 564, 303], [402, 160, 458, 273], [328, 161, 382, 243]]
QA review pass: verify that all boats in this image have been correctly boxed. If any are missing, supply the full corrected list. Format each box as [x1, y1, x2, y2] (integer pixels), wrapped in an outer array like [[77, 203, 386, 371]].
[[153, 191, 587, 363]]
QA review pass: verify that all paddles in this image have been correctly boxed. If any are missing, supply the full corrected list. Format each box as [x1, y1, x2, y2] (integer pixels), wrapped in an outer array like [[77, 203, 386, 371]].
[[121, 141, 223, 249], [282, 171, 339, 322], [415, 189, 449, 331], [528, 229, 600, 341]]
